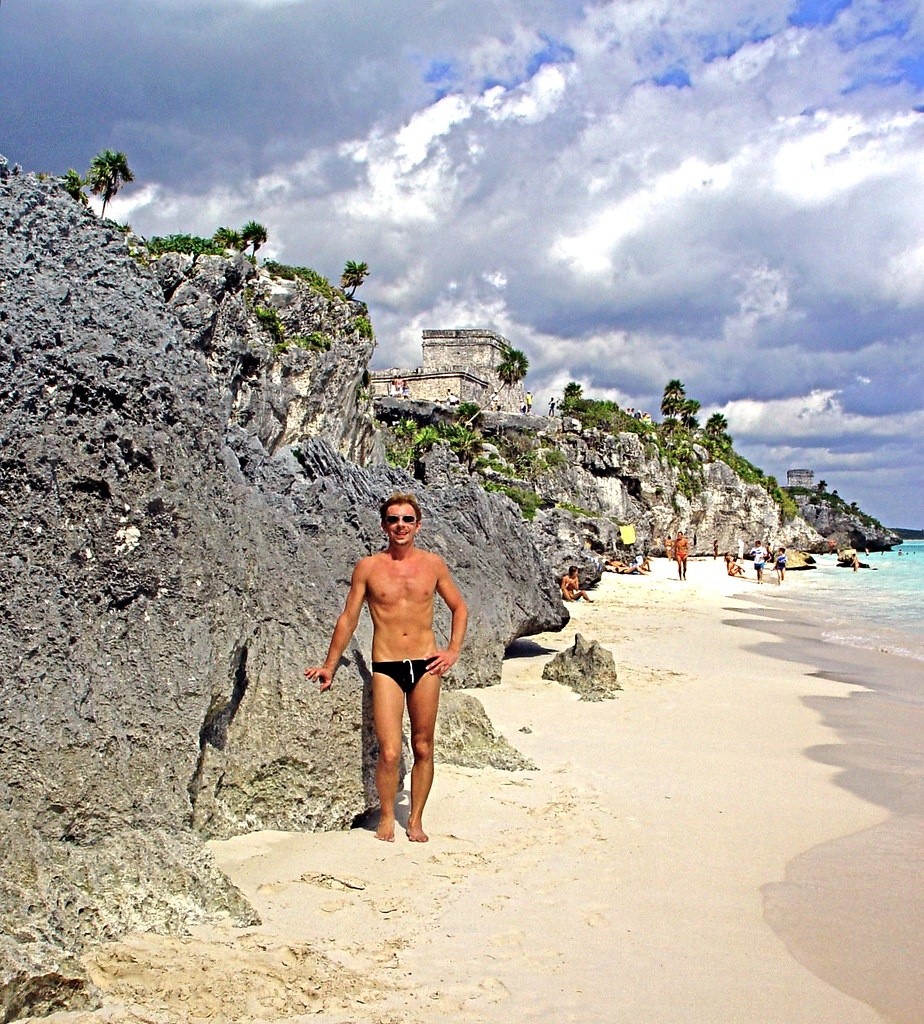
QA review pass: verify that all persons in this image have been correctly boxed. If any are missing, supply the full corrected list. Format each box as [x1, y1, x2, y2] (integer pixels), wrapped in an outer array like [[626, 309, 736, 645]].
[[820, 546, 824, 555], [881, 549, 915, 556], [518, 391, 534, 417], [606, 558, 652, 574], [749, 541, 768, 583], [548, 397, 555, 416], [850, 554, 859, 571], [447, 392, 455, 405], [390, 379, 409, 398], [865, 547, 869, 556], [669, 531, 690, 579], [724, 551, 746, 576], [305, 492, 466, 842], [561, 566, 595, 603], [766, 542, 773, 563], [625, 407, 652, 426], [713, 540, 718, 560], [775, 547, 787, 586], [489, 390, 500, 411], [655, 535, 674, 561]]
